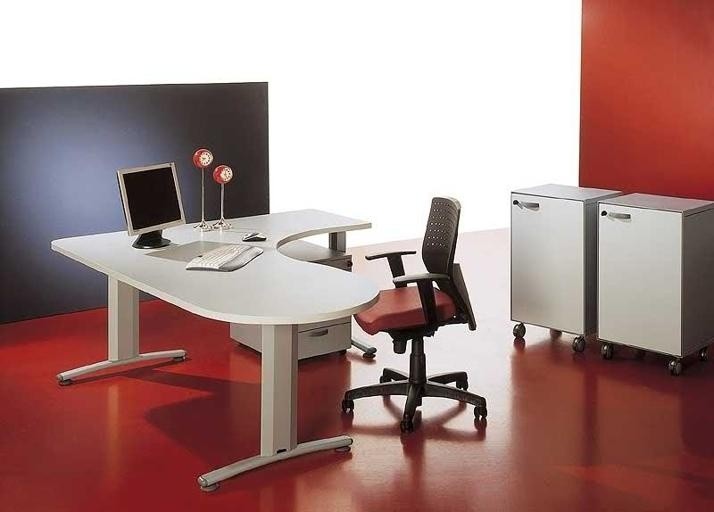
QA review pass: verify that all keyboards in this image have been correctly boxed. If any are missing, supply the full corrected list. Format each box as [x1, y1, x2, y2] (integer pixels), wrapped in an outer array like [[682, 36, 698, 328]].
[[185, 244, 264, 272]]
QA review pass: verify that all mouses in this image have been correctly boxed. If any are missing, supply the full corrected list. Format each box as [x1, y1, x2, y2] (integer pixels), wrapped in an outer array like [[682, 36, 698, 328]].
[[241, 232, 266, 241]]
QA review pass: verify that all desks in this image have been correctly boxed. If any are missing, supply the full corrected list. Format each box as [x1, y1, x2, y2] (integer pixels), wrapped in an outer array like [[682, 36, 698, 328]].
[[48, 208, 381, 494]]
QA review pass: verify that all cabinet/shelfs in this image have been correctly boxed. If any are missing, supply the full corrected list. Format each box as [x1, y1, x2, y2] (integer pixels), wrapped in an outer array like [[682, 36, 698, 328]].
[[508, 184, 622, 354], [228, 239, 352, 361], [596, 193, 714, 375]]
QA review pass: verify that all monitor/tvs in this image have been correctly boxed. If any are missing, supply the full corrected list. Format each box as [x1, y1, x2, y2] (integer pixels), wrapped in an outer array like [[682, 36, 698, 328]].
[[116, 162, 186, 249]]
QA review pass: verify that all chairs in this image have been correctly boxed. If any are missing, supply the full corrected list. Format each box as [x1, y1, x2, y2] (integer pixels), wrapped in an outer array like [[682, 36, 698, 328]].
[[340, 194, 487, 432]]
